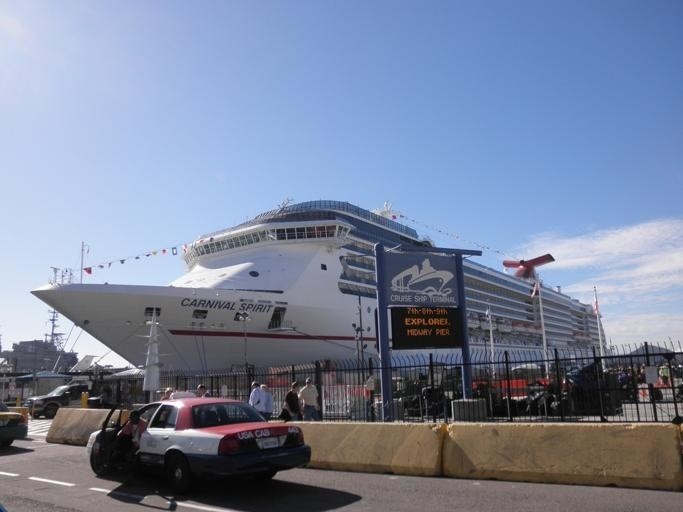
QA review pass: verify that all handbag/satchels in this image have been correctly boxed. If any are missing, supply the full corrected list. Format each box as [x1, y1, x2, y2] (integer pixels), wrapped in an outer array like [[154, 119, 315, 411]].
[[278, 409, 291, 421]]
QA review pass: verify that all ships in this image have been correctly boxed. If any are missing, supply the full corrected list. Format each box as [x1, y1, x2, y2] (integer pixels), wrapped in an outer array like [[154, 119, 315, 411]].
[[28, 199, 617, 392], [0, 266, 116, 402]]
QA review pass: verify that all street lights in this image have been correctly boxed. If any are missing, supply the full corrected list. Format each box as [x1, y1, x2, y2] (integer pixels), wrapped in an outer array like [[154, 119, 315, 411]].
[[482, 303, 497, 378]]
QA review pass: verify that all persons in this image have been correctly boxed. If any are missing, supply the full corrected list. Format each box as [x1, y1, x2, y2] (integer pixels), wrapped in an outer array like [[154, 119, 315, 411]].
[[618, 362, 646, 385], [659, 363, 683, 385], [197, 384, 210, 397], [249, 381, 272, 420], [284, 377, 321, 421], [159, 387, 172, 400], [116, 411, 148, 488]]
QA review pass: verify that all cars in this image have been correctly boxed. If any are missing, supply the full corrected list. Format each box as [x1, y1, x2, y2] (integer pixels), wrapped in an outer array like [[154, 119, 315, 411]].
[[1, 401, 29, 454], [84, 390, 315, 490]]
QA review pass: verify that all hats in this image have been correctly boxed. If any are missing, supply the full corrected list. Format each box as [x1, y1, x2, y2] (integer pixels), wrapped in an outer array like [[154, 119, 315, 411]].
[[292, 382, 298, 387], [128, 410, 140, 418], [306, 378, 311, 382]]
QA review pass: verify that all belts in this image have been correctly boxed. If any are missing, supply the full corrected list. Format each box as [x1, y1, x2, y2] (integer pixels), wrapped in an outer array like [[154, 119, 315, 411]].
[[305, 406, 315, 407]]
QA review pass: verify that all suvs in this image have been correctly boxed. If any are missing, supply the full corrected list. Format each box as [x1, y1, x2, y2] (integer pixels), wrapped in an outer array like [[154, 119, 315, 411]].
[[24, 383, 107, 423]]
